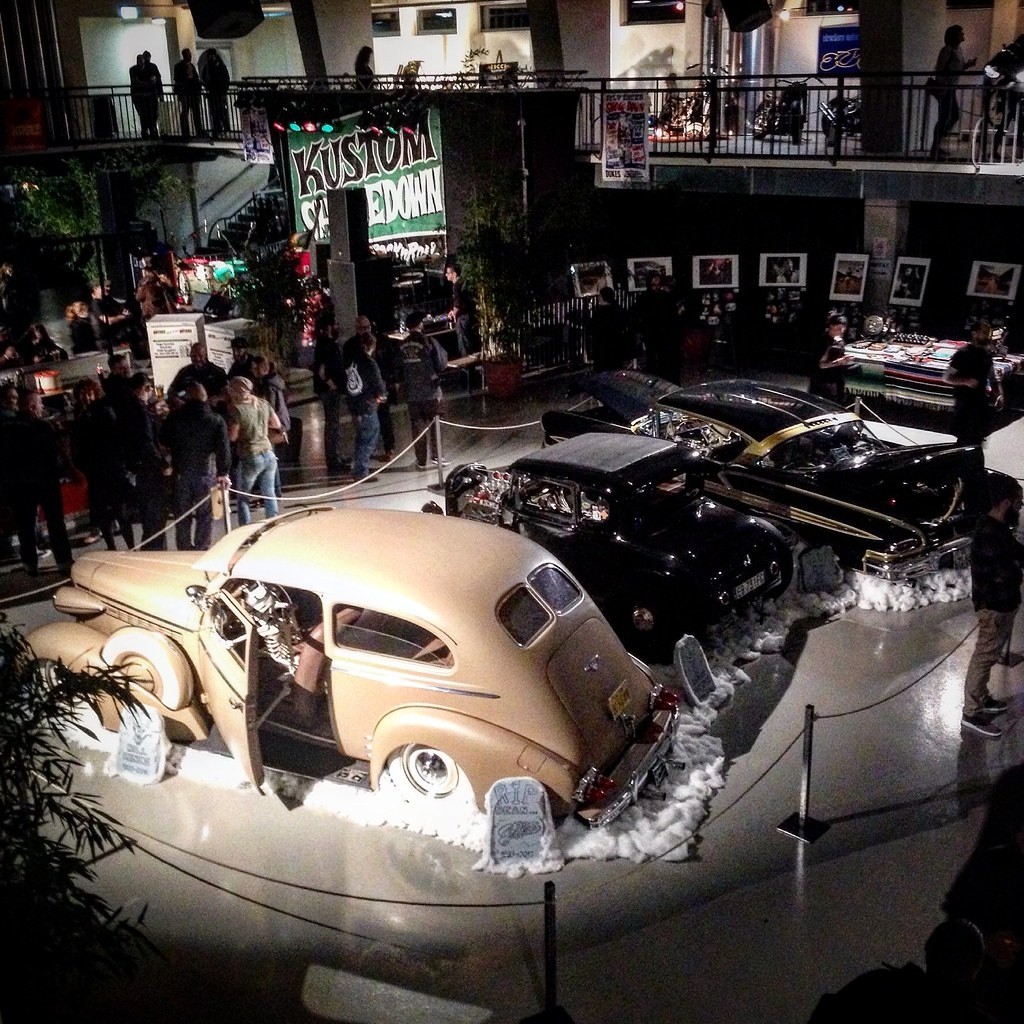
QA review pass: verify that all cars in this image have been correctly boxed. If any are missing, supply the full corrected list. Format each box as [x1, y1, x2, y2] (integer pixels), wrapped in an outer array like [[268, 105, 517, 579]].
[[28, 508, 679, 826], [539, 370, 1017, 587], [422, 433, 795, 665]]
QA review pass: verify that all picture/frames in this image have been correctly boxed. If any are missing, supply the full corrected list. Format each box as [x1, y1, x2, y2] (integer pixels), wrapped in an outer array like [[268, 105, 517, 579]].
[[759, 253, 808, 287], [571, 261, 614, 297], [691, 254, 740, 289], [965, 260, 1022, 300], [888, 256, 931, 308], [627, 256, 672, 294], [829, 253, 869, 303]]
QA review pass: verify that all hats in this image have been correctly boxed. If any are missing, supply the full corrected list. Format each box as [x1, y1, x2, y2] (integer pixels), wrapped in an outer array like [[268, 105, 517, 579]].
[[230, 375, 253, 396], [355, 316, 371, 328]]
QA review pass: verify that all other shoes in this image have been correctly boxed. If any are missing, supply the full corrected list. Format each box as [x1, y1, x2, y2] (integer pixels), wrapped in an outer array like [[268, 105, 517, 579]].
[[416, 459, 426, 469], [342, 456, 353, 465], [369, 468, 381, 475], [340, 467, 353, 473], [378, 453, 395, 462], [84, 532, 100, 544], [432, 456, 450, 466], [355, 474, 377, 483]]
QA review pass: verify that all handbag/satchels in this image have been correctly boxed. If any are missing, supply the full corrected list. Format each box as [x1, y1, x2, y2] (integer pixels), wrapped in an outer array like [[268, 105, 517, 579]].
[[420, 335, 446, 387], [346, 362, 364, 395], [208, 483, 233, 521]]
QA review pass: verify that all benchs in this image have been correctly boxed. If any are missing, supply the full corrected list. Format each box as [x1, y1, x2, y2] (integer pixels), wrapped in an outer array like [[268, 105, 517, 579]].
[[447, 348, 492, 389]]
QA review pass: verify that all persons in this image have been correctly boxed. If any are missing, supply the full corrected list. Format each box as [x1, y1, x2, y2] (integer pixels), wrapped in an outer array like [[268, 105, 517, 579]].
[[960, 474, 1024, 737], [0, 262, 470, 573], [585, 268, 687, 383], [942, 322, 1005, 448], [129, 47, 232, 140], [929, 26, 977, 159], [355, 46, 375, 88], [810, 310, 856, 406]]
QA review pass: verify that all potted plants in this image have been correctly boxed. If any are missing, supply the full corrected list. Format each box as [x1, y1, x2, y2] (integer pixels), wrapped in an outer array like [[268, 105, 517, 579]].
[[456, 155, 559, 402], [640, 163, 752, 368]]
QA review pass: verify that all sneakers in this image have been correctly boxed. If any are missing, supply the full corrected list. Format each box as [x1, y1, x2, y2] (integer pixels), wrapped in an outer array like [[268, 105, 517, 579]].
[[37, 548, 51, 559]]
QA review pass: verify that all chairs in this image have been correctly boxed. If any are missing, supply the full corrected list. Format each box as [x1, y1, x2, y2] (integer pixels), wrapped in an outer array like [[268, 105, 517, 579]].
[[258, 608, 359, 725]]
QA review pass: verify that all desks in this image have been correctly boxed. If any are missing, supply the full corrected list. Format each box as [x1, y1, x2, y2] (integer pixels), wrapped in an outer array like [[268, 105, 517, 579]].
[[383, 325, 456, 350]]
